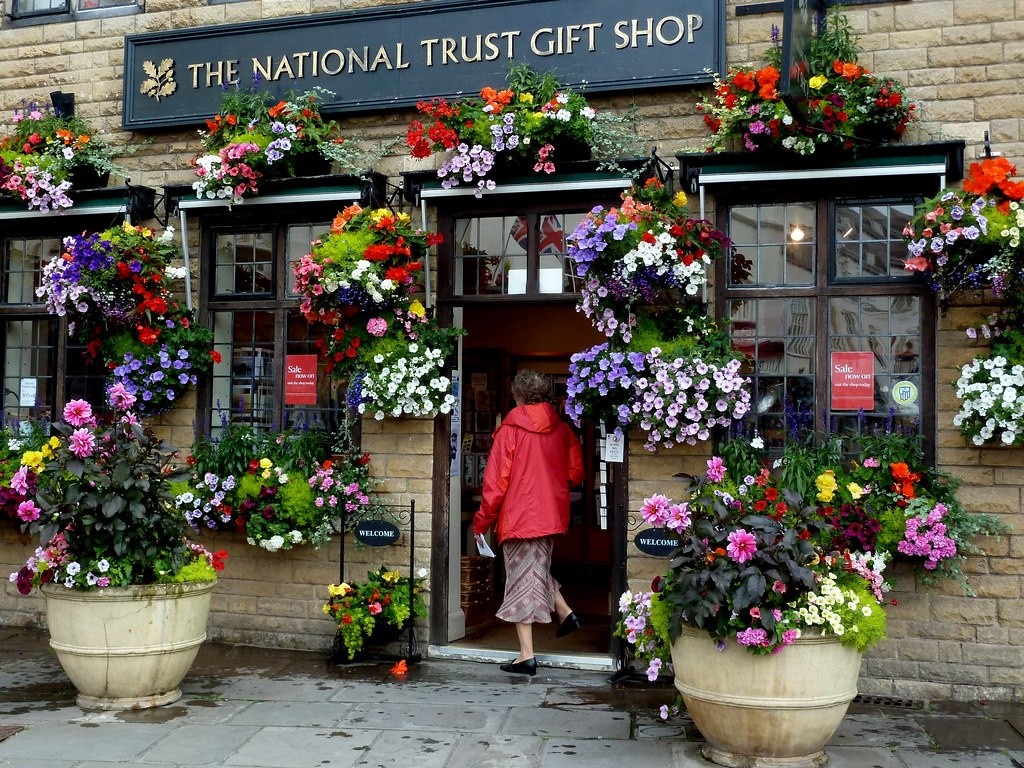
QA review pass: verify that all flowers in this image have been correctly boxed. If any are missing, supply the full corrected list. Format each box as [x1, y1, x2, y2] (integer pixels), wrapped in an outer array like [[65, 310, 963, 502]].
[[0, 96, 128, 212], [174, 392, 408, 551], [323, 564, 433, 678], [194, 66, 398, 212], [33, 220, 223, 421], [290, 204, 471, 441], [900, 156, 1024, 447], [406, 64, 658, 201], [0, 393, 46, 520], [696, 2, 940, 158], [8, 385, 230, 595], [551, 181, 757, 453], [615, 586, 681, 683], [646, 427, 1011, 657]]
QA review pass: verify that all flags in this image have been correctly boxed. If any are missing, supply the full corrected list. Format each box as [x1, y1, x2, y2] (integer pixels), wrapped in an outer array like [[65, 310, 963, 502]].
[[510, 214, 570, 266]]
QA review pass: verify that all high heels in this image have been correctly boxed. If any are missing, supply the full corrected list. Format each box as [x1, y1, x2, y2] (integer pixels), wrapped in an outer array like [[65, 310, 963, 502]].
[[500, 657, 537, 676], [556, 612, 580, 637]]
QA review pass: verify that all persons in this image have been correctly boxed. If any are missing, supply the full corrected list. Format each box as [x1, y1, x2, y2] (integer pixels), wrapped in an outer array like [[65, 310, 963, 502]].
[[471, 369, 585, 676]]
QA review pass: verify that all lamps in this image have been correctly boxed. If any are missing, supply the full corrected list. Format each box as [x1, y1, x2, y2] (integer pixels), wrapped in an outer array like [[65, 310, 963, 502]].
[[123, 178, 168, 232]]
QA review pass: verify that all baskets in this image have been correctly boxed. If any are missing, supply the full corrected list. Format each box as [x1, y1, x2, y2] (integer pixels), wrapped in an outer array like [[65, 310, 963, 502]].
[[461, 556, 494, 621]]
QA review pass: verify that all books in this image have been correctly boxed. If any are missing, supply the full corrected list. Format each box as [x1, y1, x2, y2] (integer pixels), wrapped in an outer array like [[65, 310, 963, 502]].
[[476, 533, 495, 558]]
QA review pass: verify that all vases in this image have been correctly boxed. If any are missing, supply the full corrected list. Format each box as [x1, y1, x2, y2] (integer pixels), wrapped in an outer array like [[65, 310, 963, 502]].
[[741, 121, 774, 153], [67, 159, 110, 189], [546, 131, 592, 163], [664, 611, 858, 768], [261, 137, 334, 179], [617, 672, 678, 689], [854, 107, 904, 145], [239, 168, 281, 198], [39, 578, 218, 713], [792, 135, 850, 169]]
